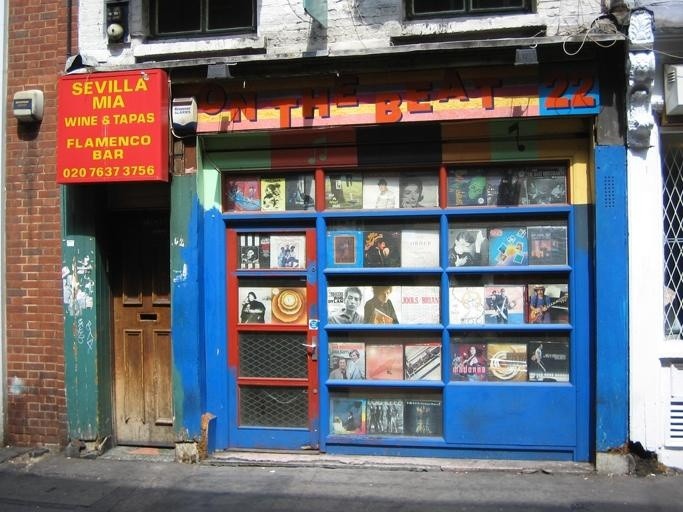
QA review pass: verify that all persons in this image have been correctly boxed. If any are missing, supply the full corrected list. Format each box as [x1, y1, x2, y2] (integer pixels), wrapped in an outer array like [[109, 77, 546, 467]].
[[368, 236, 384, 266], [448, 228, 486, 266], [461, 344, 480, 380], [368, 400, 399, 433], [328, 358, 352, 381], [240, 247, 258, 262], [531, 342, 545, 375], [333, 414, 354, 435], [275, 243, 299, 268], [485, 286, 512, 323], [239, 291, 266, 324], [365, 285, 398, 325], [348, 347, 365, 379], [344, 409, 354, 429], [373, 176, 396, 207], [530, 282, 551, 322], [399, 176, 426, 207], [414, 402, 435, 437], [380, 245, 391, 265], [329, 287, 362, 325]]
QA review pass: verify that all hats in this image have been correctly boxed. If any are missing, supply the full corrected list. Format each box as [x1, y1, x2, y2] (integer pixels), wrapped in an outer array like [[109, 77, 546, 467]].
[[533, 284, 544, 290]]
